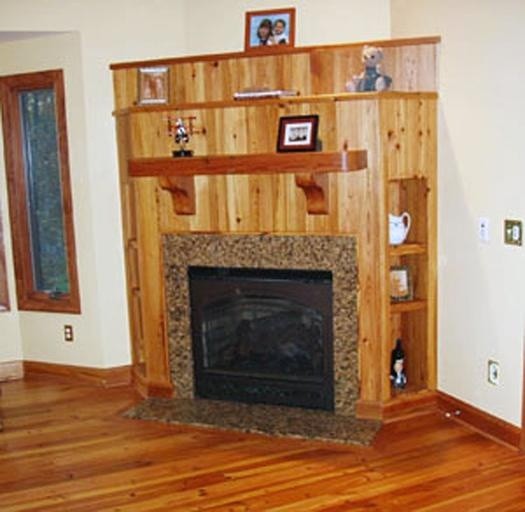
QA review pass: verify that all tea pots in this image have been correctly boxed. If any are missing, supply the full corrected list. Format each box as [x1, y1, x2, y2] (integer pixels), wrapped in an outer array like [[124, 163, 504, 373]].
[[388, 211, 412, 246]]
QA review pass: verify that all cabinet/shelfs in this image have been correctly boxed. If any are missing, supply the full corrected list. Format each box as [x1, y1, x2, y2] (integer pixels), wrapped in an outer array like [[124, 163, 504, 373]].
[[107, 33, 442, 428]]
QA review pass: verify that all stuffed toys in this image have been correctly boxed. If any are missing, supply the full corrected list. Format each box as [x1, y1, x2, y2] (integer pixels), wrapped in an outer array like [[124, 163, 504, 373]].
[[342, 42, 394, 94], [166, 117, 190, 150]]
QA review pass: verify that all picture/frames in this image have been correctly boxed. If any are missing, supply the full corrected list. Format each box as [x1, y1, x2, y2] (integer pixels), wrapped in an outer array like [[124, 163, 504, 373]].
[[276, 113, 318, 151], [243, 6, 296, 50]]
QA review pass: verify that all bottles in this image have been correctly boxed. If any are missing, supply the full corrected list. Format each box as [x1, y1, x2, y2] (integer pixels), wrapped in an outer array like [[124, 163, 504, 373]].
[[389, 337, 407, 388]]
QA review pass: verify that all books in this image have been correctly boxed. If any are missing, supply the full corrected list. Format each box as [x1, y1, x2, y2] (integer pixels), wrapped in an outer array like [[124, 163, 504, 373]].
[[390, 262, 415, 305], [233, 85, 301, 101]]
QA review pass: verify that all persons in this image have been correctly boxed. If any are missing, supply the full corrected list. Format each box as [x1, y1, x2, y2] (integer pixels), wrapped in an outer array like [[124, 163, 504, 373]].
[[271, 17, 289, 45], [254, 17, 276, 46], [389, 346, 410, 394], [152, 76, 166, 100], [142, 74, 155, 99]]
